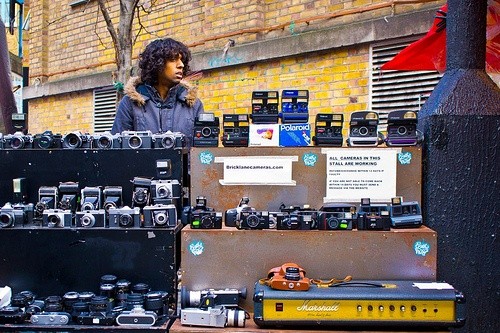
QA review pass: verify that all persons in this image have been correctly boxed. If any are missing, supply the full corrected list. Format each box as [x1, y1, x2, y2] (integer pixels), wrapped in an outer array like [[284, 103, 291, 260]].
[[110, 37, 208, 180]]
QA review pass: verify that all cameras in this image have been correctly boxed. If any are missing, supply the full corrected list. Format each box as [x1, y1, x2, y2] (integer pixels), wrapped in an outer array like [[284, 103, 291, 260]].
[[0, 91, 423, 333]]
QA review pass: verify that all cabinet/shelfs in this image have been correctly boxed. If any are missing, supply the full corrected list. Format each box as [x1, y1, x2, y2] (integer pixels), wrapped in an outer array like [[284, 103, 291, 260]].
[[1, 147, 188, 332], [168, 143, 436, 333]]
